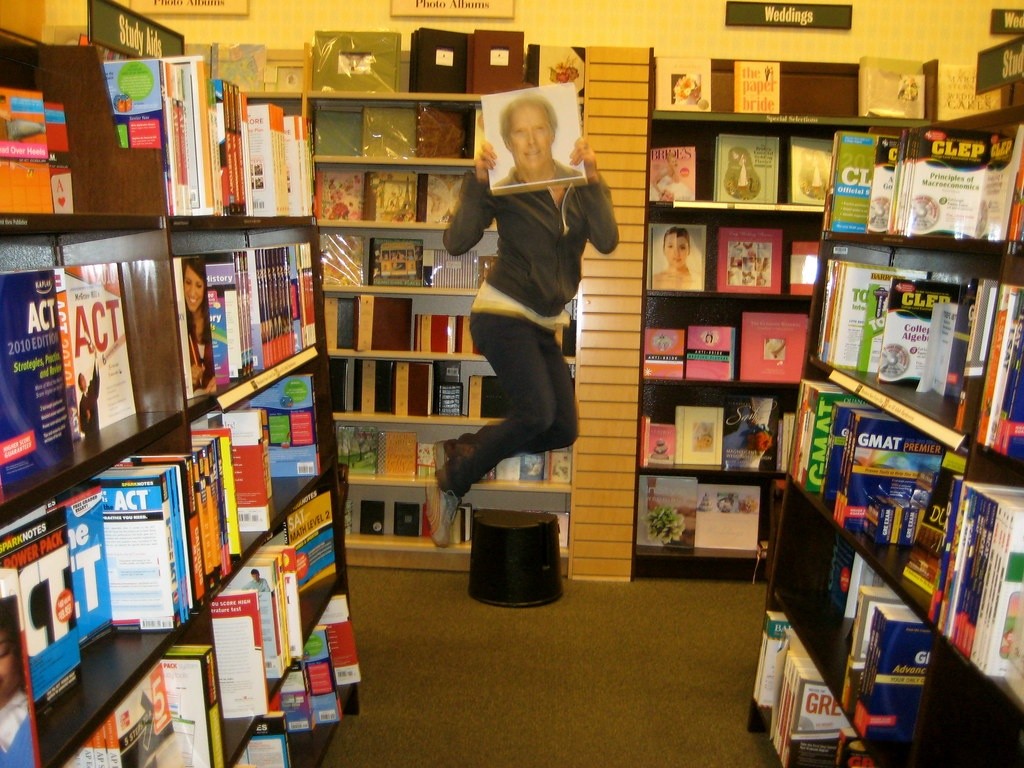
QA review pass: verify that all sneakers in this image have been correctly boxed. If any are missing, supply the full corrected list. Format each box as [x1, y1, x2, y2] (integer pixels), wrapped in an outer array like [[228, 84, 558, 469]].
[[426, 480, 462, 548], [433, 440, 450, 472]]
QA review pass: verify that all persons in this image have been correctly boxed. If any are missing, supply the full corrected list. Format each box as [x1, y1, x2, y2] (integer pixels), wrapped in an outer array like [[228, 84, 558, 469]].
[[422, 133, 624, 553]]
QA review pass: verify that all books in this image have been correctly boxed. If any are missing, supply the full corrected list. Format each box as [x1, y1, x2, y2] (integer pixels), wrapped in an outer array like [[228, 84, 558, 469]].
[[634, 40, 1022, 768], [0, 0, 585, 767]]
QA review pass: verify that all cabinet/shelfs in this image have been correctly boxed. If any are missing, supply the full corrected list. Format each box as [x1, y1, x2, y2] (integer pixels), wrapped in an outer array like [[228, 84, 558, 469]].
[[0, 27, 1024, 768]]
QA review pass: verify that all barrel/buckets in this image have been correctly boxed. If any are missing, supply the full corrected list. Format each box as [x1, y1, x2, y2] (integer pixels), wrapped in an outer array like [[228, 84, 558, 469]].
[[467, 509, 564, 606]]
